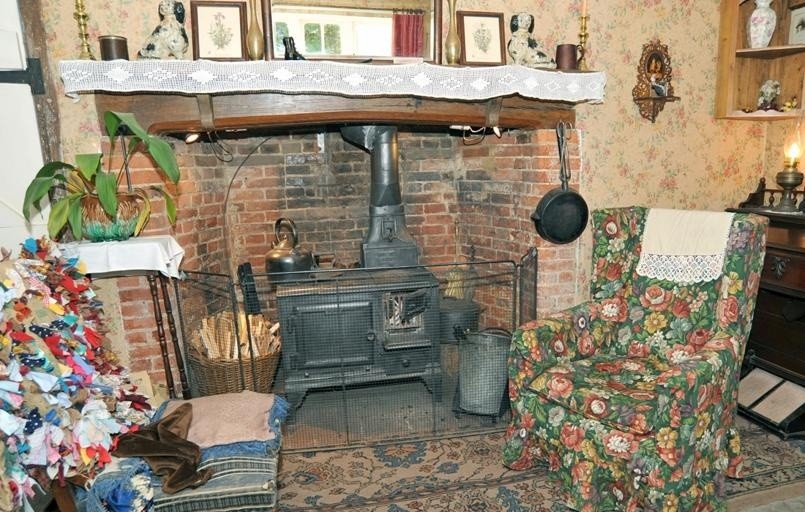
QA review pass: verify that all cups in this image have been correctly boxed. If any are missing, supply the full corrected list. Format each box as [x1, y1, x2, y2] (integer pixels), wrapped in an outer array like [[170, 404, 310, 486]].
[[99, 35, 128, 61], [556, 43, 584, 73]]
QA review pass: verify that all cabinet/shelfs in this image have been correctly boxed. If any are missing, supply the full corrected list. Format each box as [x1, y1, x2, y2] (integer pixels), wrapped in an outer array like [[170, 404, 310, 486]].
[[724, 178, 805, 386], [55, 235, 193, 399], [714, 0, 804, 120]]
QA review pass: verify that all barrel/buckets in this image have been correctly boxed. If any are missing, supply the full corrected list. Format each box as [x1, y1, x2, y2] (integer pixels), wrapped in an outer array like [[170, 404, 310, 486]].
[[458, 330, 511, 415]]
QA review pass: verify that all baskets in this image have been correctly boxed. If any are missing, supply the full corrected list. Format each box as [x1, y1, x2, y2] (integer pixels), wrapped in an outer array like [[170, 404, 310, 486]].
[[187, 312, 282, 398]]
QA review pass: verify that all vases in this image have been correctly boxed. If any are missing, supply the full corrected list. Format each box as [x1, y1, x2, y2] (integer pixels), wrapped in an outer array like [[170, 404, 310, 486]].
[[245, 0, 265, 60], [746, 0, 777, 47]]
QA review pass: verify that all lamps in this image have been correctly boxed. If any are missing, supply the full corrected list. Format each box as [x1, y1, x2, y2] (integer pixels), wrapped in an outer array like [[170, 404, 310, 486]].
[[185, 132, 201, 144], [491, 125, 506, 140], [771, 143, 804, 213]]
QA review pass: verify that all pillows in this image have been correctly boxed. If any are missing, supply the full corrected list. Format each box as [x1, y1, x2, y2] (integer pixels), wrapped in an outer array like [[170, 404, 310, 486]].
[[147, 391, 291, 461]]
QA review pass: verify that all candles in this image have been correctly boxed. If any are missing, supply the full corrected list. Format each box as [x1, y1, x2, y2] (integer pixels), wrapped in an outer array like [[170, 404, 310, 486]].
[[581, 0, 587, 15]]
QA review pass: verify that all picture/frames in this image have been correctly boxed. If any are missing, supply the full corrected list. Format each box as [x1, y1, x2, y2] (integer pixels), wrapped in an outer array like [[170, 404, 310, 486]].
[[786, 3, 805, 44], [457, 11, 507, 66], [189, 0, 247, 61]]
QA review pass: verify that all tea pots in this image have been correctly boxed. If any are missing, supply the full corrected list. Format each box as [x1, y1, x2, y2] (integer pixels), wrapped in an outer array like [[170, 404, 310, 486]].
[[265, 217, 312, 285]]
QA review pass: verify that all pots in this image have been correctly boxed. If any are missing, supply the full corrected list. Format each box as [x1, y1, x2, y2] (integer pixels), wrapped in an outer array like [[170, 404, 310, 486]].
[[439, 296, 480, 343], [531, 123, 590, 244]]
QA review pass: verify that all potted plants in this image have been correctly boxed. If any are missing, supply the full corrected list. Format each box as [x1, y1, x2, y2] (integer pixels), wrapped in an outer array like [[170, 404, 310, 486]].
[[23, 110, 182, 243]]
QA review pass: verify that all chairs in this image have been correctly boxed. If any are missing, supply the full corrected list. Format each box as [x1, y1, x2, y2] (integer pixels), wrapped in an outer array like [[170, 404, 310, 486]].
[[501, 206, 769, 511]]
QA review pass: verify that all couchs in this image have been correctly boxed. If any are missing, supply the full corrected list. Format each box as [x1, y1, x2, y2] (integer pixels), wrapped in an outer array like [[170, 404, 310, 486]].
[[0, 239, 286, 512]]
